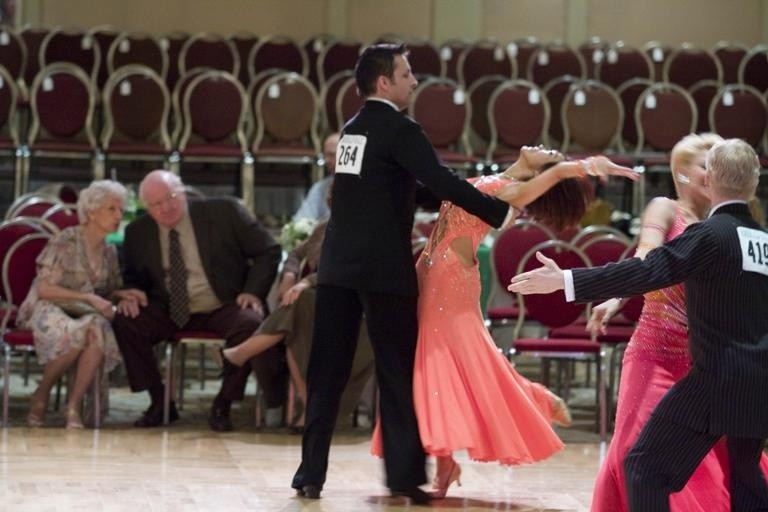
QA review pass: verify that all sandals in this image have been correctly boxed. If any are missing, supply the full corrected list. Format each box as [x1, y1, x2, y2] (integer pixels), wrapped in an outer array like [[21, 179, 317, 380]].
[[28, 393, 47, 426], [64, 406, 83, 431]]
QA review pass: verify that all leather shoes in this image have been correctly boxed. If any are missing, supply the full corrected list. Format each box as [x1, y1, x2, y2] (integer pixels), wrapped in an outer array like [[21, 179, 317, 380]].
[[298, 485, 320, 498], [211, 402, 232, 431], [135, 401, 177, 427], [546, 389, 571, 427]]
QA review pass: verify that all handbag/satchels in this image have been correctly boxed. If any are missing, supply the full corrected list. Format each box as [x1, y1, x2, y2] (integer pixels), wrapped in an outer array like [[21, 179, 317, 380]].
[[56, 300, 117, 320]]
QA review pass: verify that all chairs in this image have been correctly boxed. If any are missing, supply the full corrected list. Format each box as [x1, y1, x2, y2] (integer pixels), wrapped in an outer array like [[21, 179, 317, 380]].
[[2, 181, 647, 444], [2, 20, 767, 216]]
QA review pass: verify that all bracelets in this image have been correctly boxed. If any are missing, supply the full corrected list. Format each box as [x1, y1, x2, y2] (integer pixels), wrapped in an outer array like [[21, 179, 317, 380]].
[[576, 155, 605, 178], [615, 295, 626, 307]]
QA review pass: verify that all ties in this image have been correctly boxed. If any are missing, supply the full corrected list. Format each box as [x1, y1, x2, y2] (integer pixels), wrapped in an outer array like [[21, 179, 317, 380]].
[[168, 230, 190, 329]]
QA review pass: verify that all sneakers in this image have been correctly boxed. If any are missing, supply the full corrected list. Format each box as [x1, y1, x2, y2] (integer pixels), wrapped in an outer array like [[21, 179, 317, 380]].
[[393, 488, 430, 500]]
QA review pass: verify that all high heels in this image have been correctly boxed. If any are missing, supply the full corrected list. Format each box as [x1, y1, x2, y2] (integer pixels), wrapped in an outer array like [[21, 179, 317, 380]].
[[213, 347, 240, 378], [427, 462, 461, 499]]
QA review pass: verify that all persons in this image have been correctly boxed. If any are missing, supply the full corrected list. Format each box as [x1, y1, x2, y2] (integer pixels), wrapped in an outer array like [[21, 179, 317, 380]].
[[370, 142, 642, 498], [293, 133, 339, 222], [113, 171, 283, 431], [218, 182, 375, 432], [508, 137, 768, 512], [289, 41, 525, 503], [14, 179, 148, 430], [586, 132, 768, 511]]
